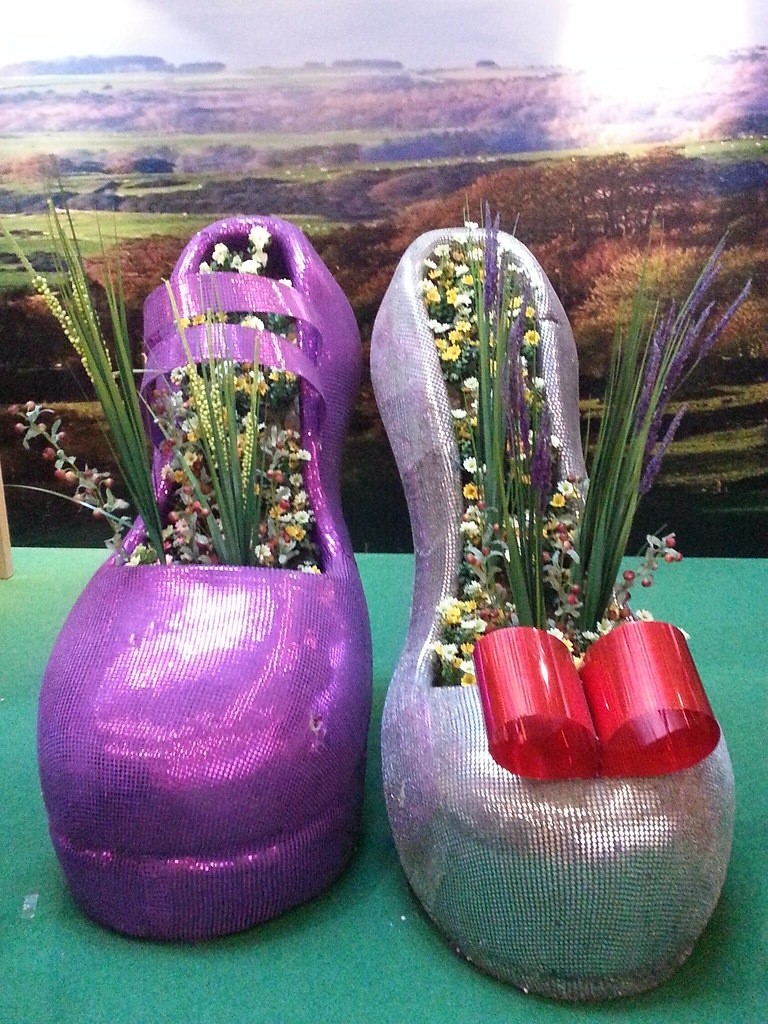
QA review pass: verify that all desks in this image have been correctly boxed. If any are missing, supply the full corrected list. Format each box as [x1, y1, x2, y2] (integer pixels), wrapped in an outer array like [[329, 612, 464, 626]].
[[2, 547, 768, 1024]]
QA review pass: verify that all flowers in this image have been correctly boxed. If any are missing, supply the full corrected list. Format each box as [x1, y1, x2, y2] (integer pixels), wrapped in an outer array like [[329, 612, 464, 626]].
[[420, 190, 753, 689], [0, 157, 320, 573]]
